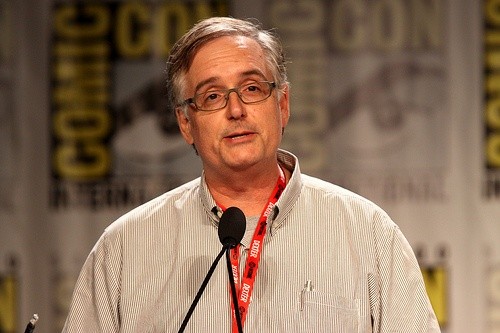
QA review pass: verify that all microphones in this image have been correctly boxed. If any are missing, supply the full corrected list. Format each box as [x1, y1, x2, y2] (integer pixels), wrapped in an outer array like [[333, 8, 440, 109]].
[[177, 207, 247, 332]]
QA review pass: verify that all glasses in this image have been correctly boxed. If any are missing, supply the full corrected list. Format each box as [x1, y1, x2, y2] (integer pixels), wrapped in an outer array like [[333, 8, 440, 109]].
[[178, 79, 279, 112]]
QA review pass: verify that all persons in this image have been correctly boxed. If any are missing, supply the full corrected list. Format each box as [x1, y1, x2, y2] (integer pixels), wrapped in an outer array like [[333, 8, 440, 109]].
[[61, 17, 444, 333]]
